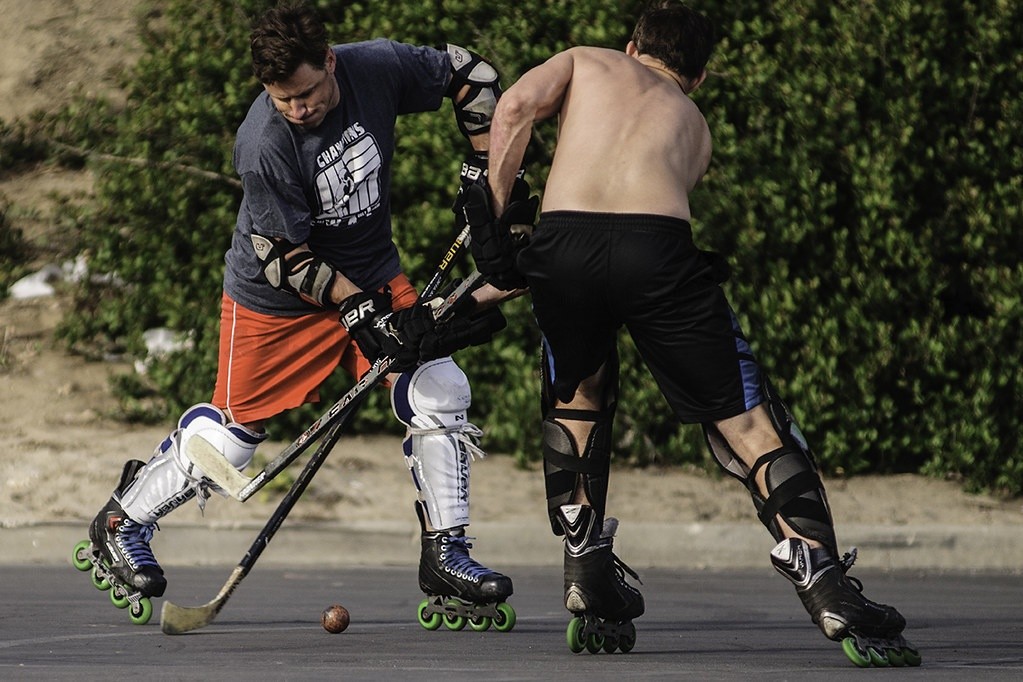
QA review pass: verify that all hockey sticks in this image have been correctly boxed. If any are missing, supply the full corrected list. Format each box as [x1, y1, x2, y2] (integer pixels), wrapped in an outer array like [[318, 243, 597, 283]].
[[158, 224, 473, 637], [181, 268, 485, 503]]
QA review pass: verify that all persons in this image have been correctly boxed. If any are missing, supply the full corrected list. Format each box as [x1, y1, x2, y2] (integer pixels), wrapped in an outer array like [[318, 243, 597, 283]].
[[463, 0, 927, 667], [72, 0, 532, 631]]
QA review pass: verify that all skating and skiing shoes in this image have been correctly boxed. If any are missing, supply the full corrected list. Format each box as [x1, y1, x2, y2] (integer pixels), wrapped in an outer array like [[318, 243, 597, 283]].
[[414, 498, 517, 633], [557, 504, 644, 656], [75, 459, 167, 626], [770, 540, 923, 668]]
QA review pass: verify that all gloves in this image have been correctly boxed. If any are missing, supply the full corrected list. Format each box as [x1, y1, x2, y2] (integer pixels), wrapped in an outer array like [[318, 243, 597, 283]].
[[461, 182, 541, 292], [386, 287, 507, 369], [451, 155, 498, 227], [337, 284, 399, 368]]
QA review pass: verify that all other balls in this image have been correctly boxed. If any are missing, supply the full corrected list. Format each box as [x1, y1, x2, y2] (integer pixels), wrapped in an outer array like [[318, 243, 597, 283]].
[[320, 604, 350, 634]]
